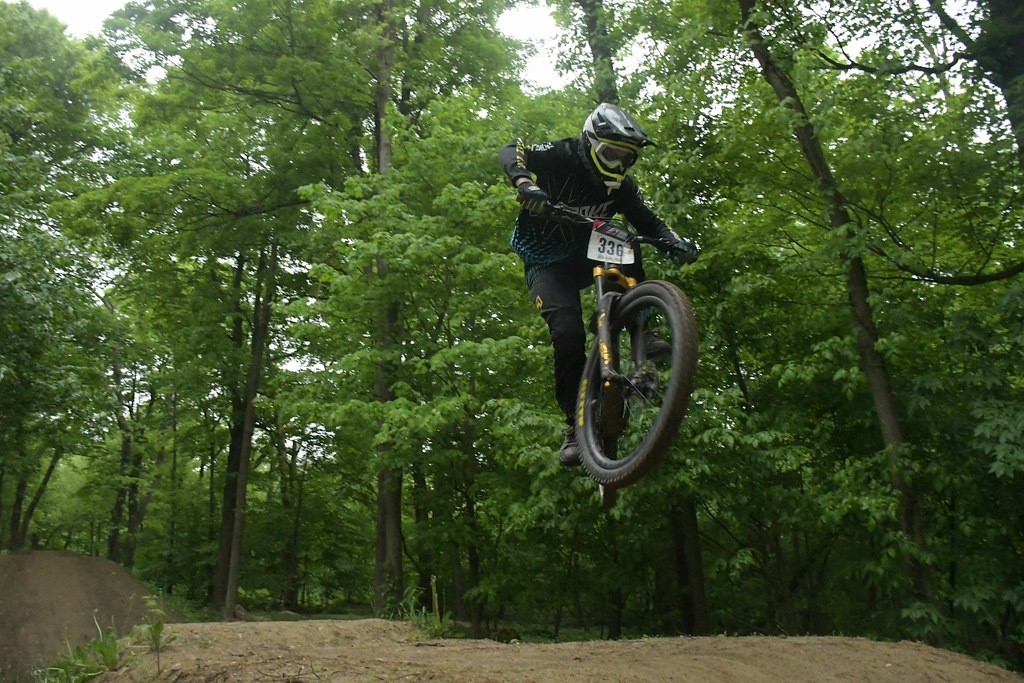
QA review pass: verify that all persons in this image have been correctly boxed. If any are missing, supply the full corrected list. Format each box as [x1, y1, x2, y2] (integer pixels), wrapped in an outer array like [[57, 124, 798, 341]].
[[497, 104, 694, 466]]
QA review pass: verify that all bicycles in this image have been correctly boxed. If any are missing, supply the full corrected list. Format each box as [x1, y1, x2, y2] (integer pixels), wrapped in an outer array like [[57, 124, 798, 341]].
[[514, 190, 704, 511]]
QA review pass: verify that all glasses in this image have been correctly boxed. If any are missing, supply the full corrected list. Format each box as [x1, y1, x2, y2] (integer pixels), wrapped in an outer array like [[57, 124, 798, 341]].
[[587, 130, 640, 169]]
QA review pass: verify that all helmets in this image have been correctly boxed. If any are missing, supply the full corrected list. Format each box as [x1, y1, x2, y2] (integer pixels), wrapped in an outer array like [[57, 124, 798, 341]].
[[579, 102, 657, 185]]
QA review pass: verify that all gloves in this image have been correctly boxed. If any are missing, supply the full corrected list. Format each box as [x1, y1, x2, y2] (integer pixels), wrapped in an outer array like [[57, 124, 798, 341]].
[[518, 182, 549, 215], [674, 240, 699, 265]]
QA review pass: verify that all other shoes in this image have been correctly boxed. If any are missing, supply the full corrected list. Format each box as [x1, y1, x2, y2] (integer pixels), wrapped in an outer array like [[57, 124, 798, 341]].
[[559, 427, 582, 467], [631, 323, 673, 361]]
